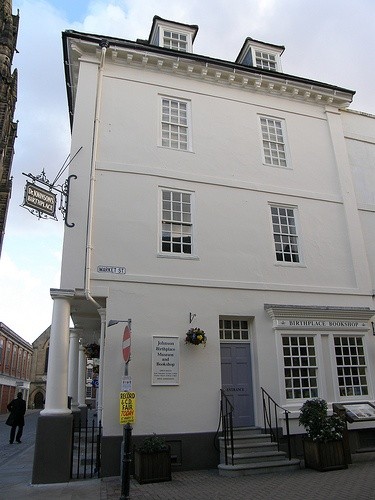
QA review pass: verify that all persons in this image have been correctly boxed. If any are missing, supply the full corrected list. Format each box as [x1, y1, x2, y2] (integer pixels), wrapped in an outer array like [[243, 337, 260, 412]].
[[5, 392, 27, 444]]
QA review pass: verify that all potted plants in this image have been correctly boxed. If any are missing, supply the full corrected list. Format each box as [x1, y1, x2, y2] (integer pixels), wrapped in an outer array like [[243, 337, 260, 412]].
[[133, 432, 172, 485], [298, 398, 348, 472]]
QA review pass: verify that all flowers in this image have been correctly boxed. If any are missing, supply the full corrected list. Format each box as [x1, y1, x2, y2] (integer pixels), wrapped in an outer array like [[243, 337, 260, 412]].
[[83, 342, 98, 360], [183, 327, 207, 351]]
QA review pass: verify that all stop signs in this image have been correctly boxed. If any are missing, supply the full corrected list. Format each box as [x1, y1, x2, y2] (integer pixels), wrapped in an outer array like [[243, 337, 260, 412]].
[[121, 325, 133, 364]]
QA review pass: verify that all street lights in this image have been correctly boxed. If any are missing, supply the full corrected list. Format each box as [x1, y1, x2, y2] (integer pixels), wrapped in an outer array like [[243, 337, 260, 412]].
[[107, 317, 132, 500]]
